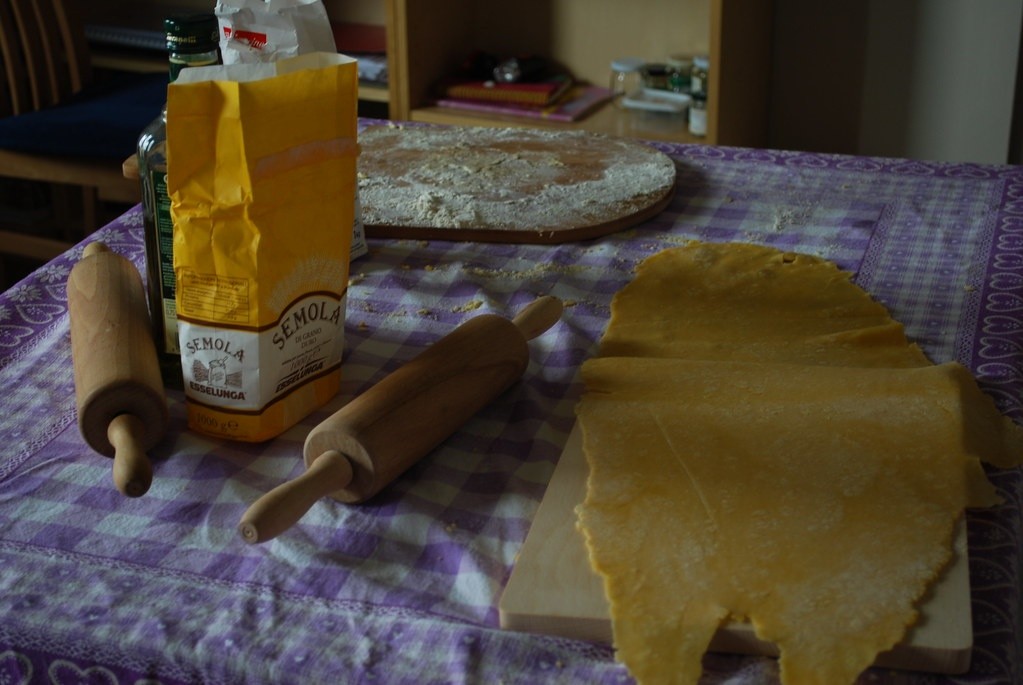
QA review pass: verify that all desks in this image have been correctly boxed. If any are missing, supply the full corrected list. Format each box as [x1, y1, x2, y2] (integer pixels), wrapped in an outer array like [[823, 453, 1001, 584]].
[[0, 113, 1023, 685]]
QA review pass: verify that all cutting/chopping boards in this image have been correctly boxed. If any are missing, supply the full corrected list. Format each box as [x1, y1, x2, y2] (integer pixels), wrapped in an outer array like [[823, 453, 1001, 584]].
[[498, 415, 973, 672], [359, 126, 679, 243]]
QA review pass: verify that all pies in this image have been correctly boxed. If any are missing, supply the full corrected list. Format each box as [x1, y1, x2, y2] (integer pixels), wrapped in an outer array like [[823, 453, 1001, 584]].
[[570, 238, 1023, 685]]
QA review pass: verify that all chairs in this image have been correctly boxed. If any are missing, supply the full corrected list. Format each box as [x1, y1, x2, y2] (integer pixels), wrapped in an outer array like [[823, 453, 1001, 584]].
[[0, 0, 172, 292]]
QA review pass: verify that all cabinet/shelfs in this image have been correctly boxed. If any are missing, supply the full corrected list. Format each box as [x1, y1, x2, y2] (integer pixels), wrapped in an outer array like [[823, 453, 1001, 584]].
[[0, 0, 766, 264]]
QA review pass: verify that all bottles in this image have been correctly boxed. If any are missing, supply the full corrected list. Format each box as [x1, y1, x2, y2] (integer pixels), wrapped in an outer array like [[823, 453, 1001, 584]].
[[137, 14, 223, 393]]
